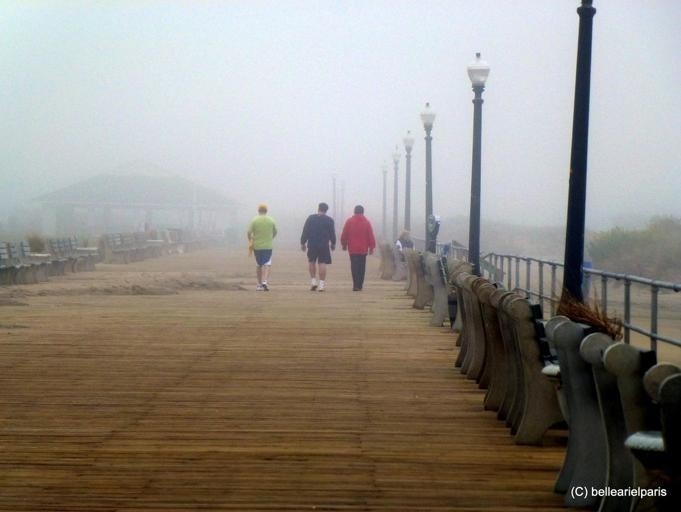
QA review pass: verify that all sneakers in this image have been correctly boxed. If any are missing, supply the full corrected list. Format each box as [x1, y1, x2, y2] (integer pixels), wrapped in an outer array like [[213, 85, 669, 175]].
[[318, 285, 325, 291], [261, 280, 269, 291], [309, 280, 317, 290], [255, 284, 263, 290]]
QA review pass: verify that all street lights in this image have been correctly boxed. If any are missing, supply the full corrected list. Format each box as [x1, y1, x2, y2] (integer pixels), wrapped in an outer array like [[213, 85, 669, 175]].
[[462, 49, 494, 277], [402, 127, 415, 233], [390, 142, 402, 244], [415, 100, 441, 254], [328, 170, 346, 226], [377, 154, 389, 239]]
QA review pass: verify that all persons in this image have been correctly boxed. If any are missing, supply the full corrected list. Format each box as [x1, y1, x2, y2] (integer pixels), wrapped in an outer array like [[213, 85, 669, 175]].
[[301, 203, 336, 292], [341, 205, 375, 291], [248, 205, 277, 291]]
